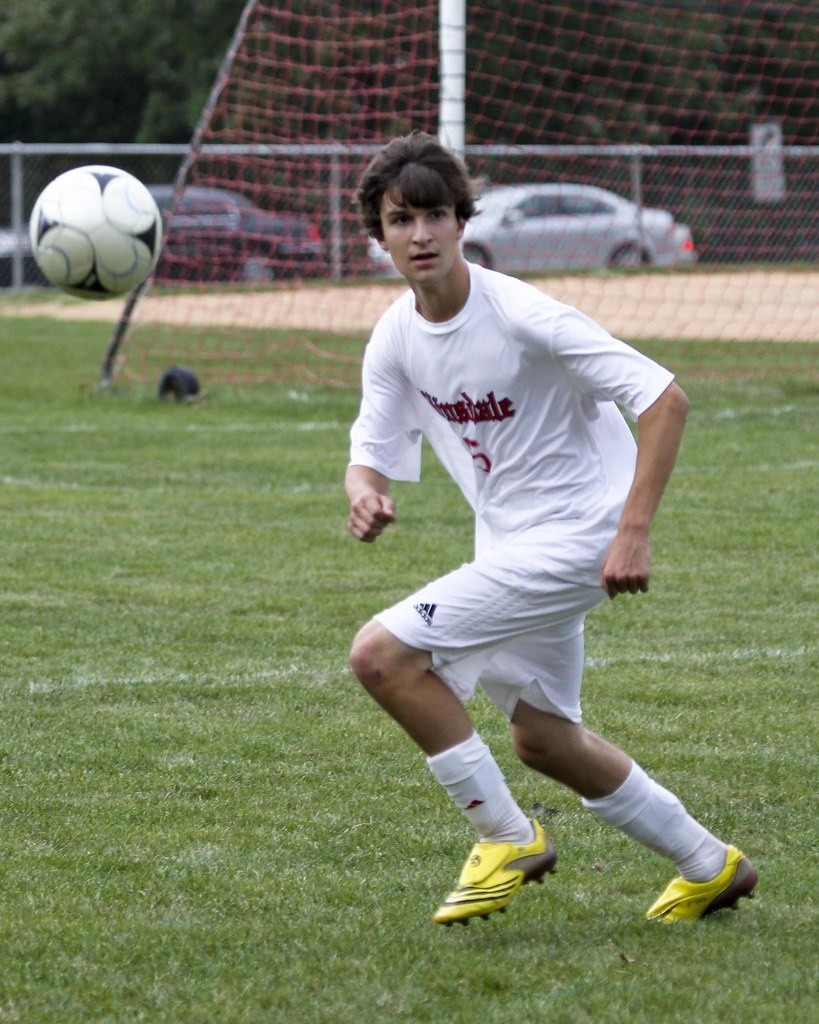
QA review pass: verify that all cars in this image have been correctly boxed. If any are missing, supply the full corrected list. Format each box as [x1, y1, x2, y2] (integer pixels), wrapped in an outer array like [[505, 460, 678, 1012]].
[[367, 183, 698, 282], [0, 185, 324, 286]]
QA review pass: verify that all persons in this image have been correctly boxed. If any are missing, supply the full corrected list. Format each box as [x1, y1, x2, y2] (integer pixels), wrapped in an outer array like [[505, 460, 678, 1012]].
[[344, 133, 759, 927]]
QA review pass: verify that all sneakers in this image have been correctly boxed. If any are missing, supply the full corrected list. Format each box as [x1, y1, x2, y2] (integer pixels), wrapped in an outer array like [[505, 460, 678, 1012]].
[[645, 845, 758, 932], [432, 818, 557, 927]]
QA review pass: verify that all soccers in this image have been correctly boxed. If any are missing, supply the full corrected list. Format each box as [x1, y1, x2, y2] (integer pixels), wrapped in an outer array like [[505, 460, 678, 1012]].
[[28, 163, 164, 302]]
